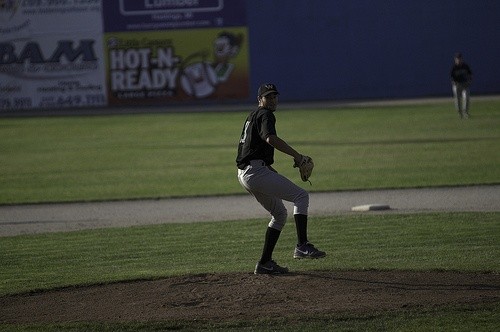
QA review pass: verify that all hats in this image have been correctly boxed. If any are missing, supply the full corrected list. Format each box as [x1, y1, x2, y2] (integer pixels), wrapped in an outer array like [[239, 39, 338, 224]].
[[258, 83, 280, 97]]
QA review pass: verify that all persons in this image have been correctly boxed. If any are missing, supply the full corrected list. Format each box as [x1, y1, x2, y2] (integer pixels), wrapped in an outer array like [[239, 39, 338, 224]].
[[450, 52, 472, 119], [236, 83, 325, 274]]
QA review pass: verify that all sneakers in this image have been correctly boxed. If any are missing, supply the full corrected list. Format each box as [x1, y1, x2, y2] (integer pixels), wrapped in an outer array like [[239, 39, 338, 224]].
[[254, 261, 288, 274], [294, 242, 326, 260]]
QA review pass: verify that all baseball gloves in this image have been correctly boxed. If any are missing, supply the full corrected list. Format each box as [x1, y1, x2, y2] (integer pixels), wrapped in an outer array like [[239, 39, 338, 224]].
[[294, 155, 314, 181]]
